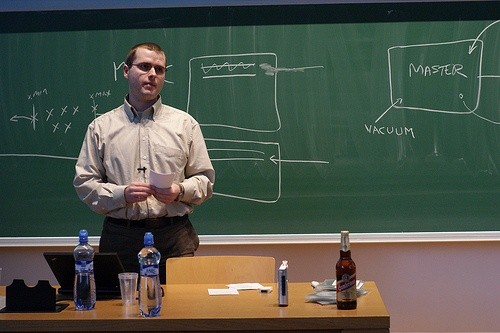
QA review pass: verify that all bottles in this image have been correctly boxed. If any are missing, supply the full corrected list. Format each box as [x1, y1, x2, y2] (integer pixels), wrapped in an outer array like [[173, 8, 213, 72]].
[[335, 230, 357, 310], [137, 232, 163, 318], [73, 229, 96, 311]]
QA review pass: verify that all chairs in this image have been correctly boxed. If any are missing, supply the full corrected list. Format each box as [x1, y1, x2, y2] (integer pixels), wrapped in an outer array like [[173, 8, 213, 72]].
[[166, 256, 275, 285]]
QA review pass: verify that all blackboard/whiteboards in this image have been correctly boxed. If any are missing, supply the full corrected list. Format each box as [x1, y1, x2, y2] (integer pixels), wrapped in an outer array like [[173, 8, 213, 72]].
[[0, 5, 500, 247]]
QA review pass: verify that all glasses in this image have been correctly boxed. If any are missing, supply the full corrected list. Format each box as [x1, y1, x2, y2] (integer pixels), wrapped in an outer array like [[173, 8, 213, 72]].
[[133, 63, 168, 75]]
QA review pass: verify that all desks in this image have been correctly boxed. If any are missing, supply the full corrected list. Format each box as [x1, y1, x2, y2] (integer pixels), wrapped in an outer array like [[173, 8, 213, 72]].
[[0, 281, 390, 333]]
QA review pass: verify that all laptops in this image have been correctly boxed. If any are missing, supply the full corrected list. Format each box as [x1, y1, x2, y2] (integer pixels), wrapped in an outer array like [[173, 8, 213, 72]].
[[43, 252, 125, 295]]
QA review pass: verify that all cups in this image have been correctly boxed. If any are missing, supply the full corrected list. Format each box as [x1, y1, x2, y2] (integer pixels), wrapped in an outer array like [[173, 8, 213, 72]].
[[118, 273, 138, 305]]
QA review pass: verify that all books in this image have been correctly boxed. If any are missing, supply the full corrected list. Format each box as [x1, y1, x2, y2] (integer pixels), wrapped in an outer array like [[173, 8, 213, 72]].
[[149, 169, 177, 190], [306, 278, 368, 308]]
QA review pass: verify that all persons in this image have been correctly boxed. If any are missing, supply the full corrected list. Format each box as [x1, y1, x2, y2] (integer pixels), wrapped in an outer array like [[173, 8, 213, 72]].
[[72, 43, 215, 283]]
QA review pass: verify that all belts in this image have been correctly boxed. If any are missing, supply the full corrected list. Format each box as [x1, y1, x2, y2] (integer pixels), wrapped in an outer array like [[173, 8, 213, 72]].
[[106, 214, 188, 228]]
